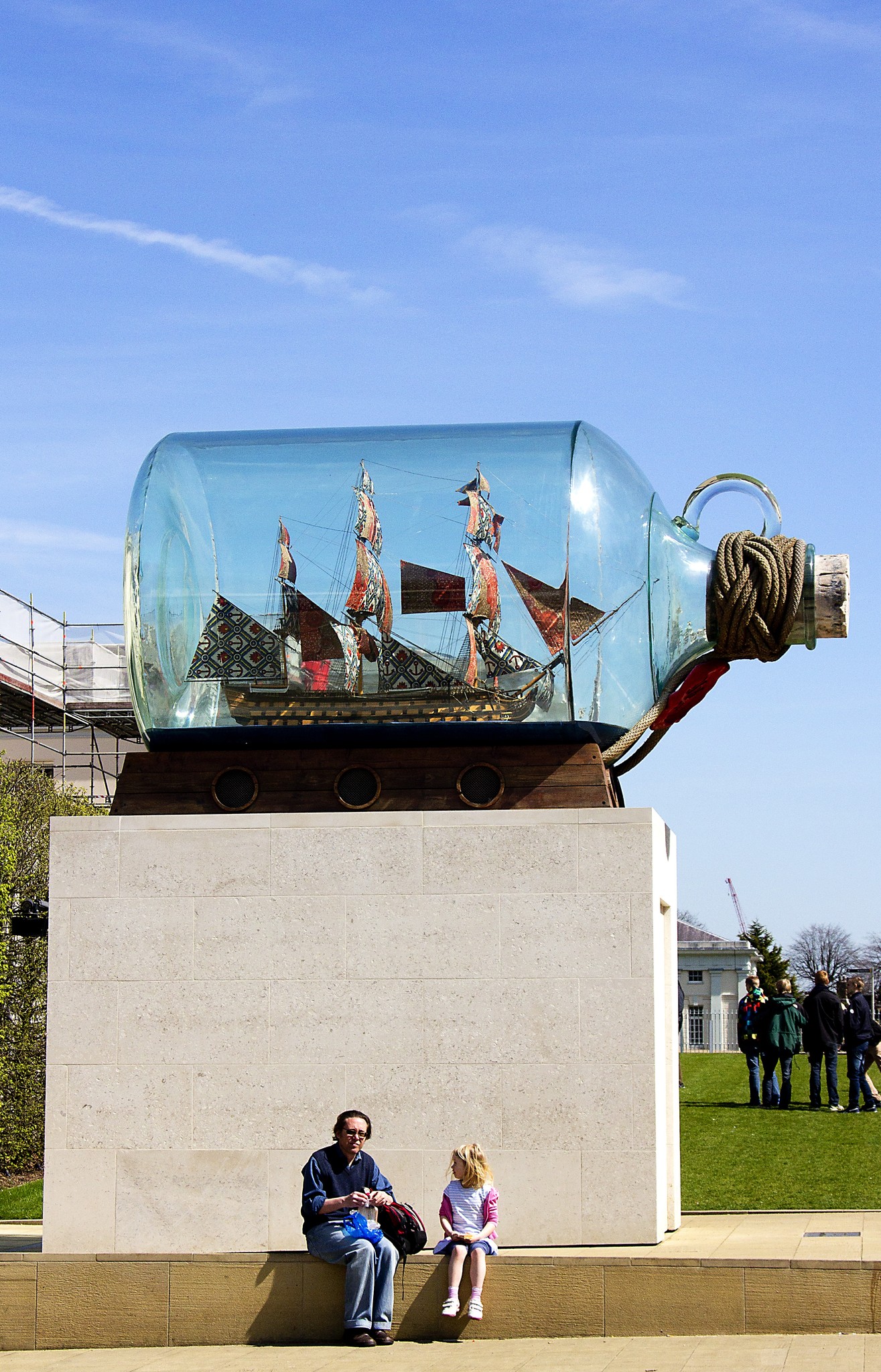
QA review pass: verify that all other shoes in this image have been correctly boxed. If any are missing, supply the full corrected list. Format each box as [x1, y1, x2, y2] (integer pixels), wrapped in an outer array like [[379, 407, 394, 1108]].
[[744, 1100, 760, 1107], [679, 1083, 685, 1088], [758, 1104, 771, 1109], [873, 1099, 881, 1107]]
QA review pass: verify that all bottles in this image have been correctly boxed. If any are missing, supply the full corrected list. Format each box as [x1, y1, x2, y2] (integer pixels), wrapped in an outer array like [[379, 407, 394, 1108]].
[[122, 420, 852, 761]]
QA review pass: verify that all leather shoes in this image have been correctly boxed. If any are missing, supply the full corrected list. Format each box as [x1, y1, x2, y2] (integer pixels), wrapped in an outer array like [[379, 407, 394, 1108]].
[[370, 1328, 393, 1344], [348, 1331, 376, 1347]]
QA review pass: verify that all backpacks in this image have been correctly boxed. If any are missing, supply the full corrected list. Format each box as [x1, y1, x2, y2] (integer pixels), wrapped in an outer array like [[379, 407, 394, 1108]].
[[365, 1187, 428, 1257]]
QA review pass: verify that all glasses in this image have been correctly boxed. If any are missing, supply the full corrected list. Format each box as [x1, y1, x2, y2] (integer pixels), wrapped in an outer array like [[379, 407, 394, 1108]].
[[340, 1126, 368, 1139]]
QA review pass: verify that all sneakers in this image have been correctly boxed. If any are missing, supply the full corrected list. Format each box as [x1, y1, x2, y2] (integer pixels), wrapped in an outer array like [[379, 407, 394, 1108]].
[[442, 1299, 460, 1317], [467, 1300, 484, 1321], [808, 1105, 821, 1109], [859, 1104, 878, 1113], [838, 1105, 860, 1113], [828, 1104, 844, 1111]]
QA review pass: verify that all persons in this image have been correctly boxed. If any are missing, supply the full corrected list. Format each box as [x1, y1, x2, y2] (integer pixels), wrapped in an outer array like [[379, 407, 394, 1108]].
[[840, 977, 877, 1114], [753, 979, 810, 1110], [737, 976, 780, 1106], [802, 970, 844, 1112], [678, 980, 685, 1089], [863, 1019, 881, 1107], [433, 1144, 498, 1320], [301, 1110, 399, 1346]]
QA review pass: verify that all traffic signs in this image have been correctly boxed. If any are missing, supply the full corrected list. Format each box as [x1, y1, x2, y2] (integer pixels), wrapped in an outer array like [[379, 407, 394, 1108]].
[[846, 969, 870, 973]]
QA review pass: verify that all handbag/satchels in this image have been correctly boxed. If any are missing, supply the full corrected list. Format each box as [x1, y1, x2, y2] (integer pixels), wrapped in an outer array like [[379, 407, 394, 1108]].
[[343, 1212, 384, 1244]]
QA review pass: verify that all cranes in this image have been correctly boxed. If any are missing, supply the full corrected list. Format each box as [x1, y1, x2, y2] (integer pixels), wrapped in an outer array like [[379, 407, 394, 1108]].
[[725, 877, 749, 935]]
